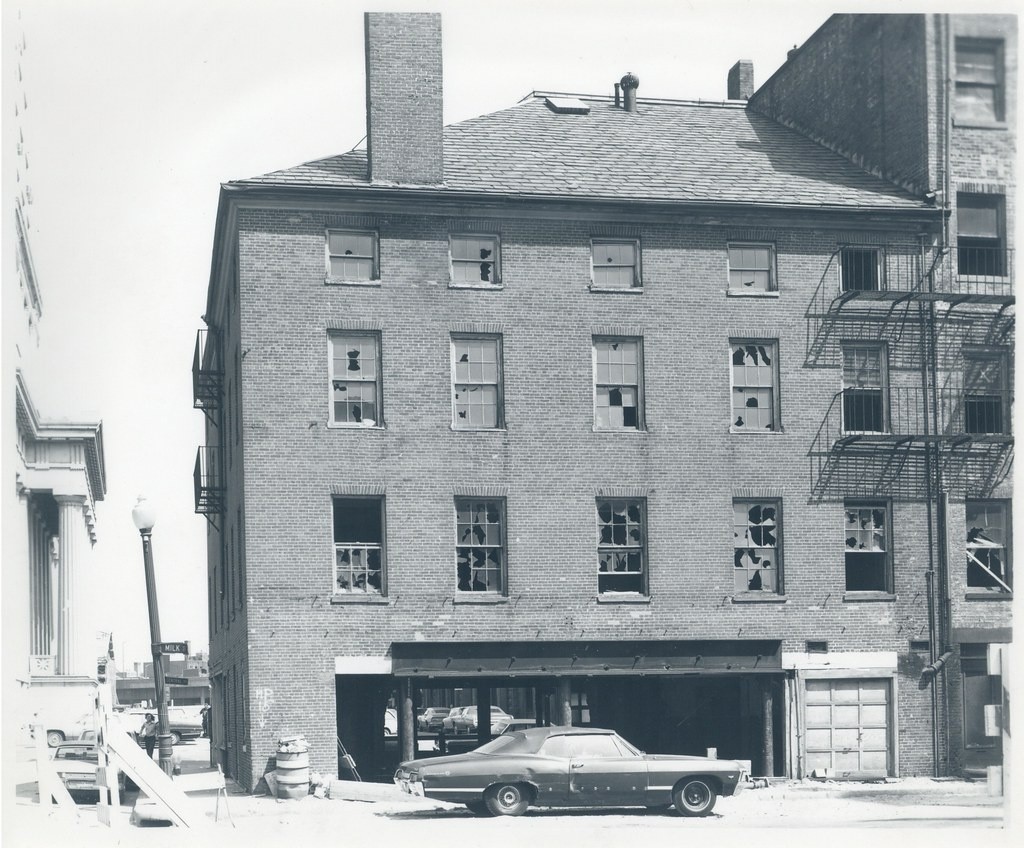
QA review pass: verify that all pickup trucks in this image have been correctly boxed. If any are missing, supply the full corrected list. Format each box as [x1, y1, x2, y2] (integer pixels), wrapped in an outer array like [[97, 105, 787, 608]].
[[432, 717, 557, 756]]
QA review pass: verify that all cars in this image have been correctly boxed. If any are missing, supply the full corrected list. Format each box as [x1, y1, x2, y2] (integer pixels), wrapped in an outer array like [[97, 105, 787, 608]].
[[50, 743, 126, 806], [394, 726, 749, 818], [79, 705, 212, 749], [384, 708, 398, 736], [417, 705, 514, 733]]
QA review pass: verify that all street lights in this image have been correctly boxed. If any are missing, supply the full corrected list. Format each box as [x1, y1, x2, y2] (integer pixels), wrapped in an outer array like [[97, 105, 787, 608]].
[[131, 494, 173, 780]]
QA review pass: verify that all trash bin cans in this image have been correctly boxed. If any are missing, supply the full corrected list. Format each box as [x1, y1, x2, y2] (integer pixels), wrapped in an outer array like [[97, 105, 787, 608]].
[[276, 747, 309, 798]]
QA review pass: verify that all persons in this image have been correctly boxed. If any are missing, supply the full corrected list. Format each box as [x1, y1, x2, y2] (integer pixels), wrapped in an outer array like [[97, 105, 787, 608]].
[[199, 702, 210, 734], [139, 713, 158, 759]]
